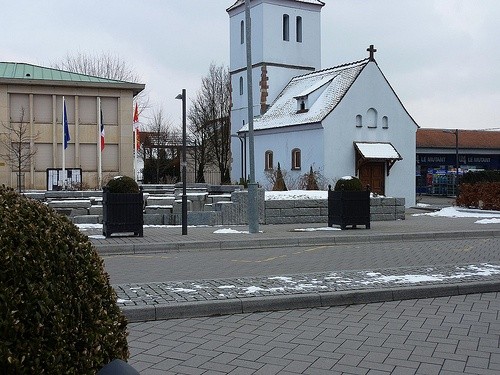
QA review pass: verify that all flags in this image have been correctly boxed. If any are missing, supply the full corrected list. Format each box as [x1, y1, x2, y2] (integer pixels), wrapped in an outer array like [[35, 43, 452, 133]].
[[64, 101, 71, 149], [100, 103, 104, 150], [133, 103, 140, 149]]
